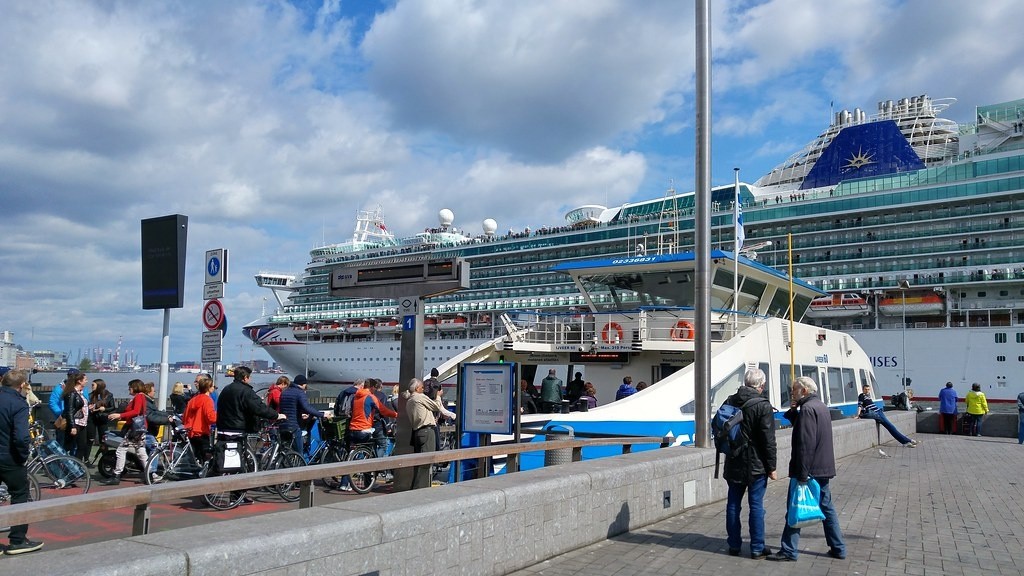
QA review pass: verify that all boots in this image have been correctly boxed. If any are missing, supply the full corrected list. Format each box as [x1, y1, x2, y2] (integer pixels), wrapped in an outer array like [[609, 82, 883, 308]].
[[135, 473, 154, 485], [100, 473, 121, 485]]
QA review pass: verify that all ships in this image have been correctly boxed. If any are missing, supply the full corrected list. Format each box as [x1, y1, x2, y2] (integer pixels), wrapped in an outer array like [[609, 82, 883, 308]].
[[241, 94, 1022, 415]]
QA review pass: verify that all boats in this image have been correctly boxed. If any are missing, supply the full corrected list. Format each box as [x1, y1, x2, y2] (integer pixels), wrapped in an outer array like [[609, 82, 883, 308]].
[[318, 322, 344, 336], [372, 317, 400, 333], [291, 326, 319, 336], [345, 319, 373, 335], [800, 290, 872, 317], [468, 311, 495, 329], [877, 289, 943, 316], [420, 316, 437, 331], [432, 313, 464, 330]]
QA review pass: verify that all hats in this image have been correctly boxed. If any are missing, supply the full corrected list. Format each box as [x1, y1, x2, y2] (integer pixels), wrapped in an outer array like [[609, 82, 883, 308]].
[[293, 375, 307, 385], [431, 367, 438, 376], [66, 368, 79, 376]]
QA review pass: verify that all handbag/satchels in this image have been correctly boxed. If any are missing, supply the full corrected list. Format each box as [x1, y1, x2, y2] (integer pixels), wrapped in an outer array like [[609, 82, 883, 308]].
[[127, 416, 145, 442], [212, 440, 245, 473], [859, 410, 881, 420], [787, 473, 828, 529]]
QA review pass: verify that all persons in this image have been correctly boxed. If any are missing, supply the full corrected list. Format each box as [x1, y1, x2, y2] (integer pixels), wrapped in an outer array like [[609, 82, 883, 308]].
[[0, 364, 456, 505], [0, 371, 45, 554], [514, 367, 648, 422], [896, 388, 933, 412], [939, 382, 959, 435], [1016, 392, 1024, 445], [852, 385, 923, 448], [764, 376, 846, 564], [964, 383, 989, 437], [711, 368, 774, 560], [291, 118, 1024, 348]]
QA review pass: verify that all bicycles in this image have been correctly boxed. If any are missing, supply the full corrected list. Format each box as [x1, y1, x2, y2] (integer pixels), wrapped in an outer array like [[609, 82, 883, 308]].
[[0, 397, 451, 533]]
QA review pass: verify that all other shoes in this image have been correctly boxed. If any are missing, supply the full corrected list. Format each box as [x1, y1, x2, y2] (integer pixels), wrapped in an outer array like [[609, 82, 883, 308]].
[[945, 431, 953, 435]]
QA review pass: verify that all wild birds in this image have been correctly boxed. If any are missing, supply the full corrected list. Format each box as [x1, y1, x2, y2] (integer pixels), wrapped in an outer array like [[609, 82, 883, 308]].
[[878, 448, 891, 459]]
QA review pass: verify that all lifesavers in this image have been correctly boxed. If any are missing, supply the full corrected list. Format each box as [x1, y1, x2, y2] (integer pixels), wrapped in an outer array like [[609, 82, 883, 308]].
[[601, 322, 623, 344], [670, 321, 695, 342]]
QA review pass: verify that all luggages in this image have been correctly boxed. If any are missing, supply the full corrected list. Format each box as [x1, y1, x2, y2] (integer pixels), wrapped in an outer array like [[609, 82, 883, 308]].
[[32, 439, 85, 488]]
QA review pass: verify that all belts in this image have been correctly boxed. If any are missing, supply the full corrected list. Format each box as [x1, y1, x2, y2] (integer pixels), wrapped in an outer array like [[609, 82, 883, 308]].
[[418, 425, 435, 430]]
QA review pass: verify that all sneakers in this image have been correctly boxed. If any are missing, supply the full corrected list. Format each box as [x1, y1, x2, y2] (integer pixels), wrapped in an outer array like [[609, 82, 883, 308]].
[[977, 433, 982, 437], [766, 549, 798, 562], [729, 547, 742, 556], [84, 461, 95, 468], [751, 545, 772, 560], [377, 471, 394, 481], [903, 439, 917, 448], [231, 495, 254, 505], [827, 549, 846, 559], [152, 472, 163, 482], [7, 537, 45, 555], [338, 485, 352, 491], [967, 432, 973, 436]]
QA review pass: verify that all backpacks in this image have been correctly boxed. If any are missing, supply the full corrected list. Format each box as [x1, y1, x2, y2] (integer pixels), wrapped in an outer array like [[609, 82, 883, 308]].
[[334, 389, 355, 416], [710, 394, 769, 458]]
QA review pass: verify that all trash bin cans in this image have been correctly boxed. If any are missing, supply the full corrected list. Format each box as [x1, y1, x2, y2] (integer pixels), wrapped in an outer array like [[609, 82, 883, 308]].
[[538, 423, 576, 468]]
